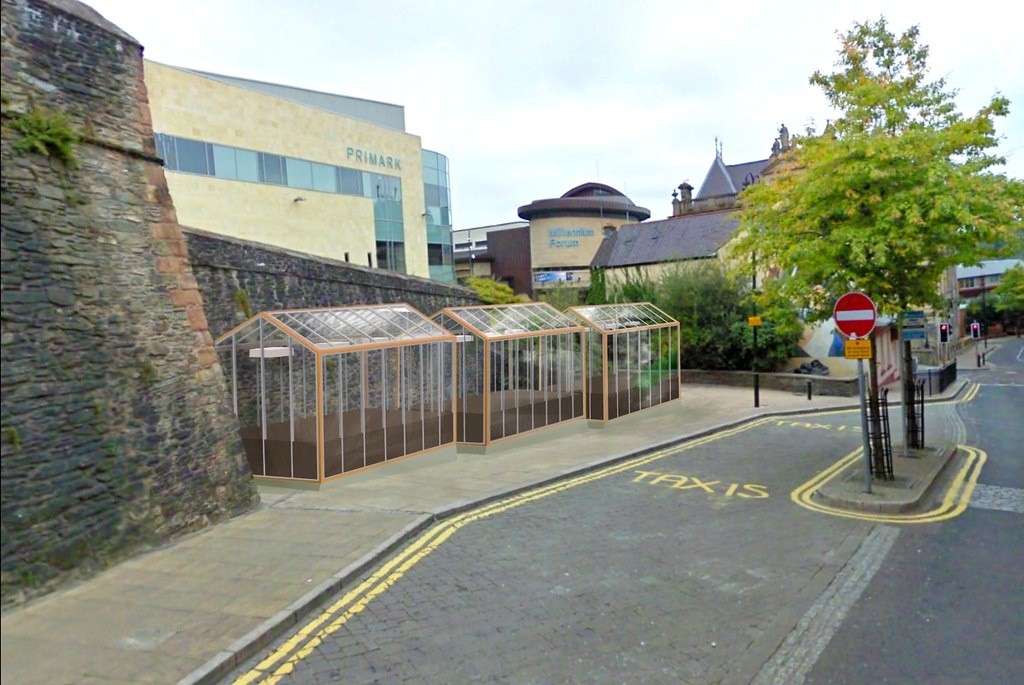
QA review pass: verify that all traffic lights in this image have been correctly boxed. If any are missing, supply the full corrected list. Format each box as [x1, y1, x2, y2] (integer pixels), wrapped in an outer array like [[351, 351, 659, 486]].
[[971, 324, 980, 339], [940, 323, 951, 343]]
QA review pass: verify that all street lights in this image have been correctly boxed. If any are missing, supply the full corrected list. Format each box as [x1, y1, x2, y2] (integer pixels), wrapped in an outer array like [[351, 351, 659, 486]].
[[742, 171, 760, 407]]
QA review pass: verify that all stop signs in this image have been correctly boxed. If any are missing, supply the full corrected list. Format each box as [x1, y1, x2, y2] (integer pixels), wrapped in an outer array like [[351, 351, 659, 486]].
[[833, 292, 875, 339]]
